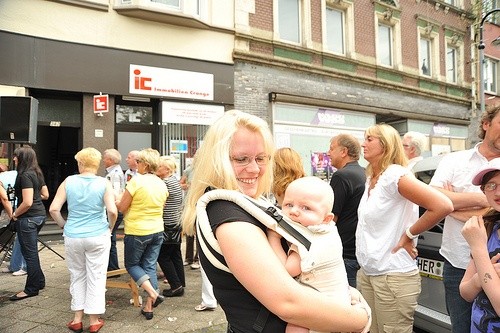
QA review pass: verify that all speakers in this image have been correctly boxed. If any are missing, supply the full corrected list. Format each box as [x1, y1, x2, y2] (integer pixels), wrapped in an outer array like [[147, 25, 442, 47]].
[[0, 96, 39, 144]]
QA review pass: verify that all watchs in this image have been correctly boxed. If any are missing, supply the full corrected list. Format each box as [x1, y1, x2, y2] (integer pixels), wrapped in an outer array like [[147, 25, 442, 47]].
[[405, 227, 419, 241]]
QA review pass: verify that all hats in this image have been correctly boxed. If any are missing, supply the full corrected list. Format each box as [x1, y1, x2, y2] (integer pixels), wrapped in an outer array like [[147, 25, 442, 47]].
[[472, 162, 500, 186]]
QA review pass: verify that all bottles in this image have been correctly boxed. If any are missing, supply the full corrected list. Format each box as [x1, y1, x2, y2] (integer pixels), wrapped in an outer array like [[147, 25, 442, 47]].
[[113, 170, 120, 192], [7, 184, 15, 201]]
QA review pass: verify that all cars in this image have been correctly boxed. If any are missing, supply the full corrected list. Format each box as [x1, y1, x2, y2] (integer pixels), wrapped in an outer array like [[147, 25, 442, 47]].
[[407, 154, 453, 333]]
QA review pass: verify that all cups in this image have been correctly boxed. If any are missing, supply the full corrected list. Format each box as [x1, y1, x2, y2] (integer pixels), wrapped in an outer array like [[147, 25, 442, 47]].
[[129, 296, 142, 305]]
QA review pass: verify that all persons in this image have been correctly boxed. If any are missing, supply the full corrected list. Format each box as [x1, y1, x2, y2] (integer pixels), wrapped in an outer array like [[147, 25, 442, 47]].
[[189, 102, 500, 333], [148, 155, 221, 310], [117, 148, 170, 319], [3, 146, 49, 301], [265, 176, 352, 333], [94, 149, 127, 277], [122, 150, 141, 186], [0, 165, 33, 275], [47, 146, 118, 333]]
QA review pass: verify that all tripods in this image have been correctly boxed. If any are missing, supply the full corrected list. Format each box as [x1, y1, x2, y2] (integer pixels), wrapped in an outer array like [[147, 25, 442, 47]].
[[0, 143, 65, 266]]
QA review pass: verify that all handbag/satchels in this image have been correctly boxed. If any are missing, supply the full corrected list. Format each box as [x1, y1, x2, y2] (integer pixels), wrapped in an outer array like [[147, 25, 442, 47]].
[[163, 225, 181, 244]]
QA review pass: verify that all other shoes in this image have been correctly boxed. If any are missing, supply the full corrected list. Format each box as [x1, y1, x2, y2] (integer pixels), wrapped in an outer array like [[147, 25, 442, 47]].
[[140, 308, 153, 320], [2, 267, 11, 273], [183, 262, 189, 266], [194, 304, 217, 311], [68, 320, 83, 333], [89, 318, 104, 333], [159, 272, 165, 278], [12, 270, 27, 275], [162, 287, 184, 297], [130, 295, 143, 305], [190, 261, 200, 269], [152, 296, 165, 307], [10, 291, 36, 301]]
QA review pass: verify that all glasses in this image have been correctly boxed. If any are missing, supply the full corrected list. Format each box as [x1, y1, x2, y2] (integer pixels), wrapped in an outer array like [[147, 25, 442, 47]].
[[230, 155, 272, 167], [482, 181, 500, 192]]
